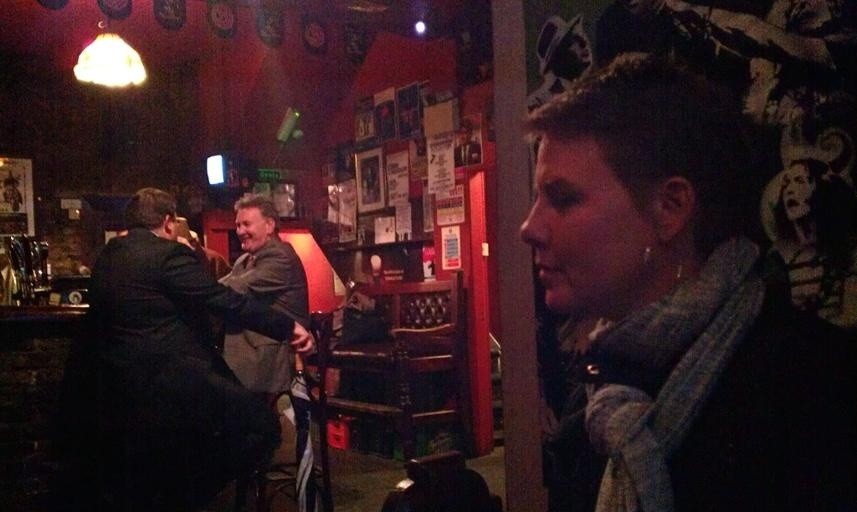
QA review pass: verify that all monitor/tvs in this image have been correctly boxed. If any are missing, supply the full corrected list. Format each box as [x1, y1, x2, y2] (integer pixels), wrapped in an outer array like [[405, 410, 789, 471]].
[[253, 180, 300, 222], [204, 151, 240, 191]]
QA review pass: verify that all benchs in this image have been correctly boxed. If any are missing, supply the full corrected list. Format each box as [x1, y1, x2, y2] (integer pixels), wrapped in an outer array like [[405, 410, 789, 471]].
[[314, 270, 463, 463]]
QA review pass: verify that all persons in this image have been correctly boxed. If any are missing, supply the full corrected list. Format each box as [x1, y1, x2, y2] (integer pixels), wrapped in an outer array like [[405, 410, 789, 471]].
[[84, 187, 317, 500], [769, 152, 854, 326], [454, 119, 481, 164], [214, 193, 311, 400], [519, 51, 857, 512]]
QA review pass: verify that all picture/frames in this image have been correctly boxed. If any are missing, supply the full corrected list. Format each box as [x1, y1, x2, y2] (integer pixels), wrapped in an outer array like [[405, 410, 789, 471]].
[[354, 143, 390, 215], [0, 153, 38, 238]]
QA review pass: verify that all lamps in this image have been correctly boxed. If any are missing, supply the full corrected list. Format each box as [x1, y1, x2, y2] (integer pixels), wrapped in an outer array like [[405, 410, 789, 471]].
[[72, 17, 148, 90]]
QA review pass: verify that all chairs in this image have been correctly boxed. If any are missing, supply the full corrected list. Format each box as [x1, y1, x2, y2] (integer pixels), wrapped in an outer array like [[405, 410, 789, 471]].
[[233, 310, 334, 511]]
